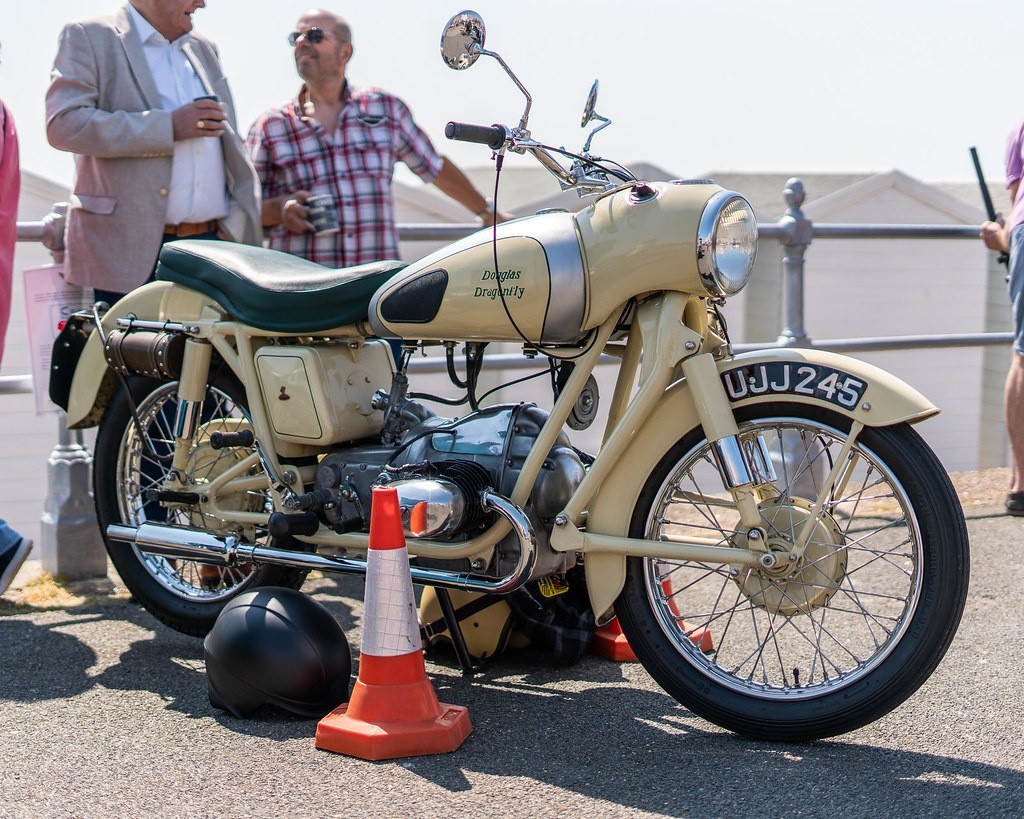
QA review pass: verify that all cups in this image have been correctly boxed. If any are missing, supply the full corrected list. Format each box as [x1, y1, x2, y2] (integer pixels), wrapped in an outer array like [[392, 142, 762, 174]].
[[302, 194, 342, 236], [193, 96, 222, 131]]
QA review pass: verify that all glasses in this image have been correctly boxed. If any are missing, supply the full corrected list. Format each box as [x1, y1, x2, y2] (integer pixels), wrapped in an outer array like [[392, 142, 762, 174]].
[[287, 28, 346, 46]]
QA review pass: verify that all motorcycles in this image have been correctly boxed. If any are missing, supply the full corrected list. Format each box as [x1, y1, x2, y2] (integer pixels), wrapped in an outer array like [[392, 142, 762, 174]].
[[45, 2, 971, 746]]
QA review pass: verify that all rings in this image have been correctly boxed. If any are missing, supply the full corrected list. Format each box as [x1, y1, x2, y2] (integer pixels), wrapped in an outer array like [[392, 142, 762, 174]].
[[196, 120, 204, 128]]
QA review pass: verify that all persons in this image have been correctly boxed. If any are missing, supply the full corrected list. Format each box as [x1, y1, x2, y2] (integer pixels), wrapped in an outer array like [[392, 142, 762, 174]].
[[979, 122, 1024, 516], [243, 7, 517, 371], [44, 0, 263, 312], [1, 96, 34, 600]]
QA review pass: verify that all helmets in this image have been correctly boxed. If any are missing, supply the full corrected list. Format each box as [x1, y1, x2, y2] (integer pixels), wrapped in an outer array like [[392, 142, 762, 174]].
[[205, 584, 353, 722], [419, 584, 516, 663]]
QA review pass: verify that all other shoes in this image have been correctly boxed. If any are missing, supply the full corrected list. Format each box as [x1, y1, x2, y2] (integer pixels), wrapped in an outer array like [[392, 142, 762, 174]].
[[1004, 489, 1024, 516], [0, 537, 34, 596], [201, 565, 253, 586]]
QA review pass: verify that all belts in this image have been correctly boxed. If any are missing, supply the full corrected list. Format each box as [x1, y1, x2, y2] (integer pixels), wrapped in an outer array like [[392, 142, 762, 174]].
[[164, 220, 219, 237]]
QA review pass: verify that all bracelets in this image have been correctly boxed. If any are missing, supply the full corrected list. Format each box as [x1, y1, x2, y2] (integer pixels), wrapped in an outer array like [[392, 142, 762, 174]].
[[474, 197, 494, 224]]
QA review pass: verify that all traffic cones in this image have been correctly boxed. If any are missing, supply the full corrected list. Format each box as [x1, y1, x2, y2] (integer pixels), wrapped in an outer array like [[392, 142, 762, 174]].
[[594, 558, 714, 662], [313, 484, 473, 763]]
